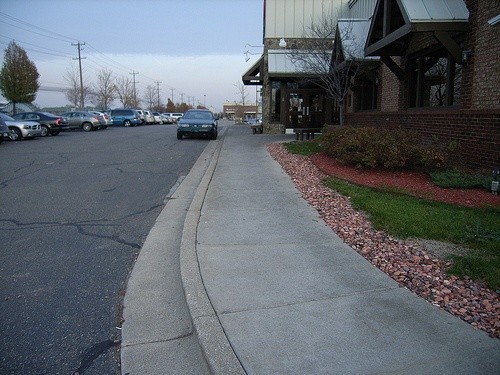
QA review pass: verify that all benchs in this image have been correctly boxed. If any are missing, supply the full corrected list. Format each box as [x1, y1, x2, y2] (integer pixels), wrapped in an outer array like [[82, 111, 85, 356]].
[[293, 128, 323, 140], [251, 124, 262, 134]]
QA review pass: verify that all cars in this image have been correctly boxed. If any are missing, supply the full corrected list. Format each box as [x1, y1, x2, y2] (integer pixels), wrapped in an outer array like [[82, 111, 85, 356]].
[[106, 108, 184, 128], [246, 119, 258, 125], [176, 109, 218, 139], [9, 112, 70, 136], [88, 110, 113, 130], [0, 111, 43, 141], [57, 111, 103, 132]]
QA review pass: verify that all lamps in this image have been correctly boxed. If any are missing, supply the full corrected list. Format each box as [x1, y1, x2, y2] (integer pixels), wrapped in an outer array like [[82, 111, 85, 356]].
[[244, 43, 264, 54], [279, 32, 287, 47], [462, 49, 472, 63], [245, 50, 262, 62]]
